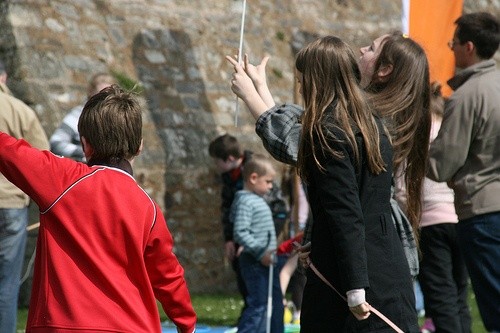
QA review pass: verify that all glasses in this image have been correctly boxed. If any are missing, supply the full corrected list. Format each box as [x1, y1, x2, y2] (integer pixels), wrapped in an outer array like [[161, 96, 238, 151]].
[[447, 40, 464, 48]]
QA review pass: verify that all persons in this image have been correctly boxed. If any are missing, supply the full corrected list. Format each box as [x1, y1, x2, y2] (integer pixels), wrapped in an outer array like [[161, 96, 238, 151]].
[[0, 63, 118, 333], [0, 86, 197, 333], [209, 10, 499, 333]]
